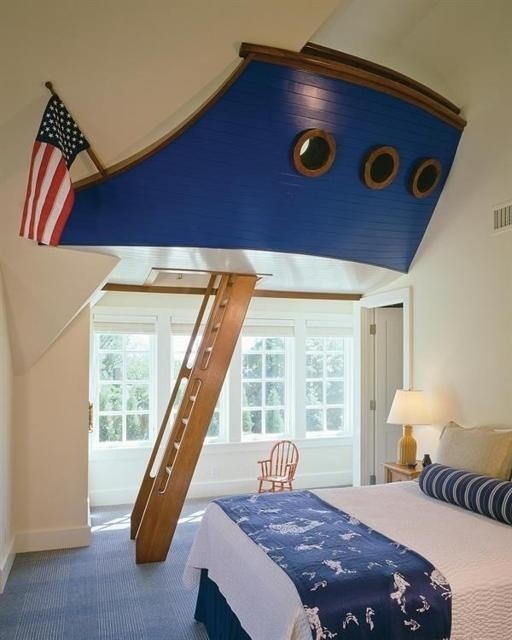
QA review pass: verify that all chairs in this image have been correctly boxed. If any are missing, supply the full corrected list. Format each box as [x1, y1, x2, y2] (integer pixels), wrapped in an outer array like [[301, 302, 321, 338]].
[[257, 441, 298, 494]]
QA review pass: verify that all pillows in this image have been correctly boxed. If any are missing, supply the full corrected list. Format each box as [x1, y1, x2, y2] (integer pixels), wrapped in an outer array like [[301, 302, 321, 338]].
[[435, 421, 512, 481], [419, 463, 512, 525]]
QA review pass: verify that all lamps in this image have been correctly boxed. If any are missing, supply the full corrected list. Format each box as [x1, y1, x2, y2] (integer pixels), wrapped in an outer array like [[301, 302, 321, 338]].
[[386, 388, 432, 465]]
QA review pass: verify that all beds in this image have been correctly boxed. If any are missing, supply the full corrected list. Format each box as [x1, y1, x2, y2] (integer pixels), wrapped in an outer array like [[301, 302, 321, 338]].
[[182, 480, 512, 640]]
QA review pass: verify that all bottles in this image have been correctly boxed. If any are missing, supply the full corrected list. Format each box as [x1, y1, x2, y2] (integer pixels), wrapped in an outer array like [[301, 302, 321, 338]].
[[422, 454, 432, 468]]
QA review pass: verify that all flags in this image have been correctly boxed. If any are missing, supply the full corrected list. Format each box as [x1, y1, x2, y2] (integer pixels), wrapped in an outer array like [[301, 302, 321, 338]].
[[19, 91, 91, 248]]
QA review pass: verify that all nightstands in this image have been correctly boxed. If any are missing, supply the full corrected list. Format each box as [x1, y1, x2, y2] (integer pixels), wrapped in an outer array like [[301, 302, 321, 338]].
[[384, 460, 431, 483]]
